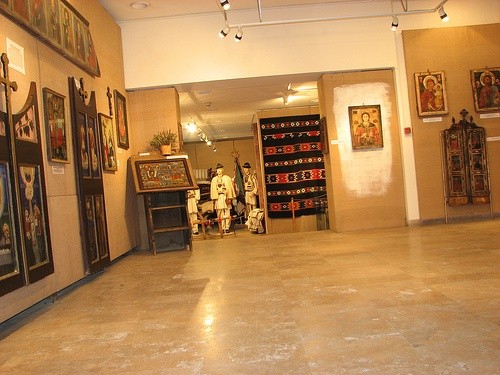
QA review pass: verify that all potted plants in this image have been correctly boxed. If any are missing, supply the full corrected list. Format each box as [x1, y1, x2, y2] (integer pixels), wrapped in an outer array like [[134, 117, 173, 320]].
[[148, 131, 180, 155]]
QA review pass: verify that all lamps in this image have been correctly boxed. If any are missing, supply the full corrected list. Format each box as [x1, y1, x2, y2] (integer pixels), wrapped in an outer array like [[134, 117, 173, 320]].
[[220, 0, 230, 10], [220, 26, 230, 38], [235, 28, 243, 42], [391, 15, 399, 31], [438, 5, 448, 22]]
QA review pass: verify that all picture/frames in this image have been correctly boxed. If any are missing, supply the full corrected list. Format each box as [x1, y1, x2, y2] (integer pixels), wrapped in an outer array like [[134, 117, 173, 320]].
[[415, 70, 449, 116], [348, 104, 384, 151], [0, 87, 128, 298], [470, 67, 500, 111], [134, 157, 195, 189]]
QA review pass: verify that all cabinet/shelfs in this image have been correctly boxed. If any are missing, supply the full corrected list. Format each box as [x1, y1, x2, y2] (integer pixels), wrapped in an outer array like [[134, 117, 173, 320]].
[[143, 193, 194, 255]]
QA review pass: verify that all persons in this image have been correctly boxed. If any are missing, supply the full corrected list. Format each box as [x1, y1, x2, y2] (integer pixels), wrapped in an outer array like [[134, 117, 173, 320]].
[[210, 163, 237, 234], [242, 162, 258, 216], [187, 189, 200, 235]]
